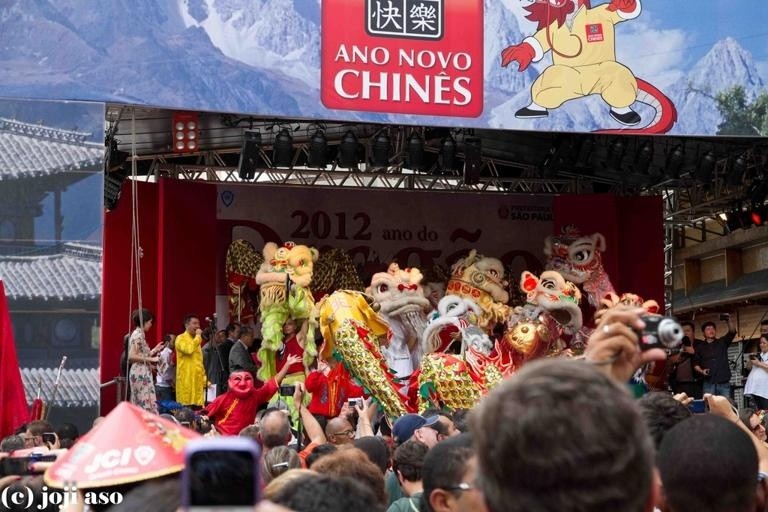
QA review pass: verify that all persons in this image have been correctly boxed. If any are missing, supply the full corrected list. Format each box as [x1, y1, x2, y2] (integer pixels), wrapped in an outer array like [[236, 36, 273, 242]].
[[0, 308, 768, 510]]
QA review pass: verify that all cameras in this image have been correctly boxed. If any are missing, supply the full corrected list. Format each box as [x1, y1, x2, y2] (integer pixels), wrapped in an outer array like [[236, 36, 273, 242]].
[[347, 398, 363, 409], [636, 315, 685, 347], [720, 315, 730, 322], [688, 399, 708, 416], [281, 385, 295, 396], [749, 352, 761, 361], [42, 432, 55, 444]]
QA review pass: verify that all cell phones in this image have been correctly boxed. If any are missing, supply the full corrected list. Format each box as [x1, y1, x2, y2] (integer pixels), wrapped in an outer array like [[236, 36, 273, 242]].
[[182, 439, 258, 512]]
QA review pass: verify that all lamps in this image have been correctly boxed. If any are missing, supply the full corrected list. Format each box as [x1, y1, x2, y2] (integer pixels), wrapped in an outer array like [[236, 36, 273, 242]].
[[541, 134, 759, 184], [104, 139, 129, 212], [725, 197, 768, 232], [221, 116, 480, 187]]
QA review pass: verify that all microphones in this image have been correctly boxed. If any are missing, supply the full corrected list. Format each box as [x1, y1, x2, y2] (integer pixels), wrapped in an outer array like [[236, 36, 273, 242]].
[[156, 352, 161, 367], [204, 316, 213, 323], [195, 328, 208, 342], [213, 313, 220, 328]]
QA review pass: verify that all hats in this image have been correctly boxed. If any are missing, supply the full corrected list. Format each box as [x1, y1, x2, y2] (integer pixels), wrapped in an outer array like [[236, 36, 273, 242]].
[[39, 393, 212, 493], [389, 411, 442, 447]]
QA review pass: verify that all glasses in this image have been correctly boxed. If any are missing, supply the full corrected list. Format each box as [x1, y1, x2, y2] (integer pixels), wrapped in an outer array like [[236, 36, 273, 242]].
[[446, 479, 481, 493], [333, 428, 355, 436], [752, 420, 765, 431]]
